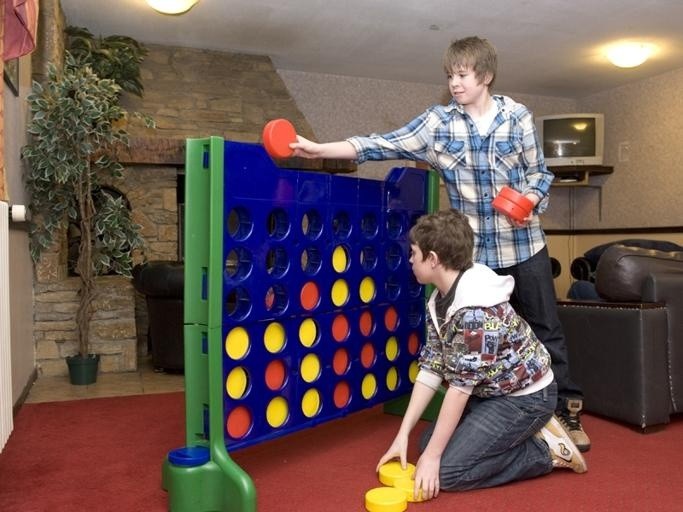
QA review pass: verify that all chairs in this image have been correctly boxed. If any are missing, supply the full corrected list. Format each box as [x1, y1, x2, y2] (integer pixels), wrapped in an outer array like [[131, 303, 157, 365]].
[[550, 240, 683, 435], [135, 261, 237, 374], [569, 239, 682, 283]]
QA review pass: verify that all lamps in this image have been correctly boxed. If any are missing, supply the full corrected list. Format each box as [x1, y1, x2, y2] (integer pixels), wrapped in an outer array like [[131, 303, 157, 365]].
[[146, 0, 197, 14], [604, 44, 650, 68]]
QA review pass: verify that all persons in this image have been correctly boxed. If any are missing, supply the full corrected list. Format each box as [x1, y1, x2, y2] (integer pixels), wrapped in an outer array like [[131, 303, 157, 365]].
[[280, 34, 594, 457], [371, 205, 591, 504]]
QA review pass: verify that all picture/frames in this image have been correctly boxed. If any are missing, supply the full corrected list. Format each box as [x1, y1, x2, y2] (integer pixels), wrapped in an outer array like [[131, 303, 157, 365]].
[[4, 58, 19, 96]]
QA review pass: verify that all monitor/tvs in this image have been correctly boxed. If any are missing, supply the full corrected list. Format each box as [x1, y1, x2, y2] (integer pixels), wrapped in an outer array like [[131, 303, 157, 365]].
[[534, 112, 604, 167]]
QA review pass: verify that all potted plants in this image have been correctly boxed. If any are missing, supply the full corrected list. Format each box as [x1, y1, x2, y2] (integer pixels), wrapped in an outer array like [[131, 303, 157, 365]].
[[63, 26, 150, 132], [23, 47, 155, 385]]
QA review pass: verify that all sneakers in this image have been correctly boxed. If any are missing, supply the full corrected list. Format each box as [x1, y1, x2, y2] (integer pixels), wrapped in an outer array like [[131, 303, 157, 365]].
[[533, 413, 587, 474], [553, 393, 591, 451]]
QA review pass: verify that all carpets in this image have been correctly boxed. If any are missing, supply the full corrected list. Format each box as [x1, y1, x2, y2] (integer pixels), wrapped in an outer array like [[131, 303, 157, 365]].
[[0, 392, 683, 512]]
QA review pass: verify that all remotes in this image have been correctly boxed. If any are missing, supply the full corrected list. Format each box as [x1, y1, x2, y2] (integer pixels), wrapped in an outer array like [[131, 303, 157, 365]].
[[559, 178, 577, 182]]
